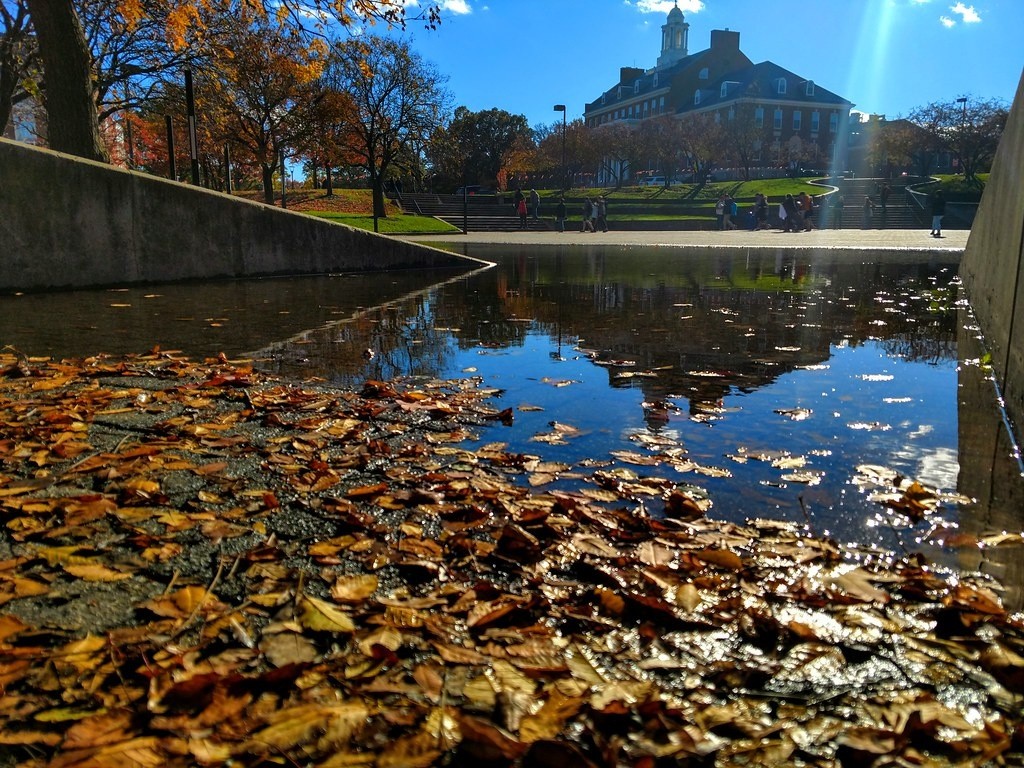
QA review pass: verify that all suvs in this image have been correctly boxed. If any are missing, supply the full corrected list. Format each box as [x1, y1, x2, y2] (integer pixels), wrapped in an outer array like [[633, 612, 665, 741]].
[[639, 177, 683, 187]]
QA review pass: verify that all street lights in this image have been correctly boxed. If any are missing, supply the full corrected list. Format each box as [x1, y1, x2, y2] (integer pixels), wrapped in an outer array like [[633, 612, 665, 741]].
[[553, 105, 566, 201], [956, 98, 969, 176]]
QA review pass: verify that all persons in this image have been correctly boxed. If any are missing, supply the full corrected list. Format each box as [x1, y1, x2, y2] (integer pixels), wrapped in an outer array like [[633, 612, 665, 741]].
[[929, 190, 946, 237], [529, 189, 539, 219], [518, 197, 528, 229], [816, 195, 830, 229], [580, 196, 609, 233], [751, 193, 771, 232], [868, 180, 880, 198], [557, 198, 567, 231], [514, 187, 524, 215], [880, 182, 890, 213], [782, 192, 813, 232], [859, 196, 873, 228], [716, 194, 738, 230], [831, 196, 845, 230]]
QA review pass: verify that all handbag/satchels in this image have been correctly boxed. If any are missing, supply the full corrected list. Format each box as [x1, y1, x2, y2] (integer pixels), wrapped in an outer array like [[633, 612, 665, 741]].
[[805, 209, 813, 218]]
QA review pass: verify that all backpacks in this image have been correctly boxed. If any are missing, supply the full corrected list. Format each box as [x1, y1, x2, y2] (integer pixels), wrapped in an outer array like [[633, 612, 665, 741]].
[[870, 202, 875, 210], [729, 200, 737, 216]]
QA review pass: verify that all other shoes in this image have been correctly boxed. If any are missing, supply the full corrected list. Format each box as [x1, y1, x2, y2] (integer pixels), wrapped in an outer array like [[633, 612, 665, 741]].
[[603, 230, 608, 232], [580, 230, 585, 232], [590, 230, 596, 233]]
